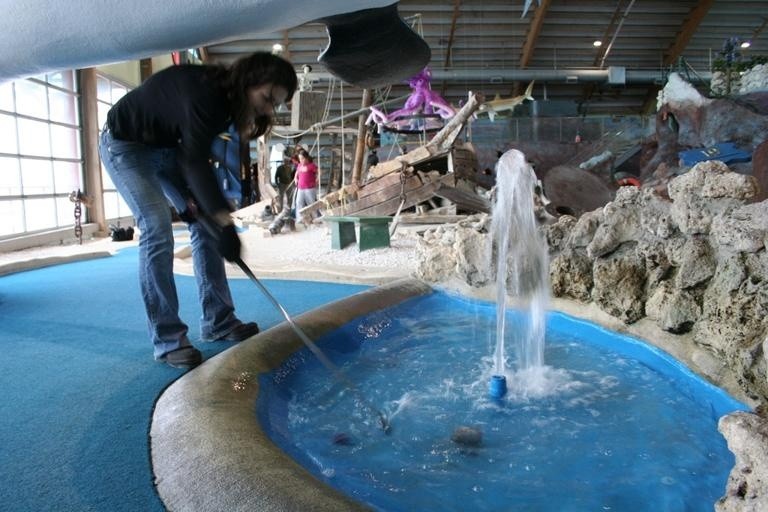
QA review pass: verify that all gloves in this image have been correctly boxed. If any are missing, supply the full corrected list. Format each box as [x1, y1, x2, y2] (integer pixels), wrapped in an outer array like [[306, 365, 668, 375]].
[[217, 224, 240, 262]]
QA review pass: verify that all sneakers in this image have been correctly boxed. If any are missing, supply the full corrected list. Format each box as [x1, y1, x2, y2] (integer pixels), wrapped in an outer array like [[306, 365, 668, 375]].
[[219, 322, 259, 342], [156, 346, 201, 369]]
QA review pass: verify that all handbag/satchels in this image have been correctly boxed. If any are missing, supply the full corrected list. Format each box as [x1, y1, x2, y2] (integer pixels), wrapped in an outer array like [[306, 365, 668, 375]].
[[112, 227, 134, 241]]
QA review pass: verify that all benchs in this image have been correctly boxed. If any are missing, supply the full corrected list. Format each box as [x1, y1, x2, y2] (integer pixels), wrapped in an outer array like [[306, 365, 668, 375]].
[[323, 215, 393, 252]]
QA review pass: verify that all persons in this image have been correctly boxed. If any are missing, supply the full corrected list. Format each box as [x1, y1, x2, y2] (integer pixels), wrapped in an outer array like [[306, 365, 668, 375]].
[[98, 49, 299, 370], [274, 144, 322, 230]]
